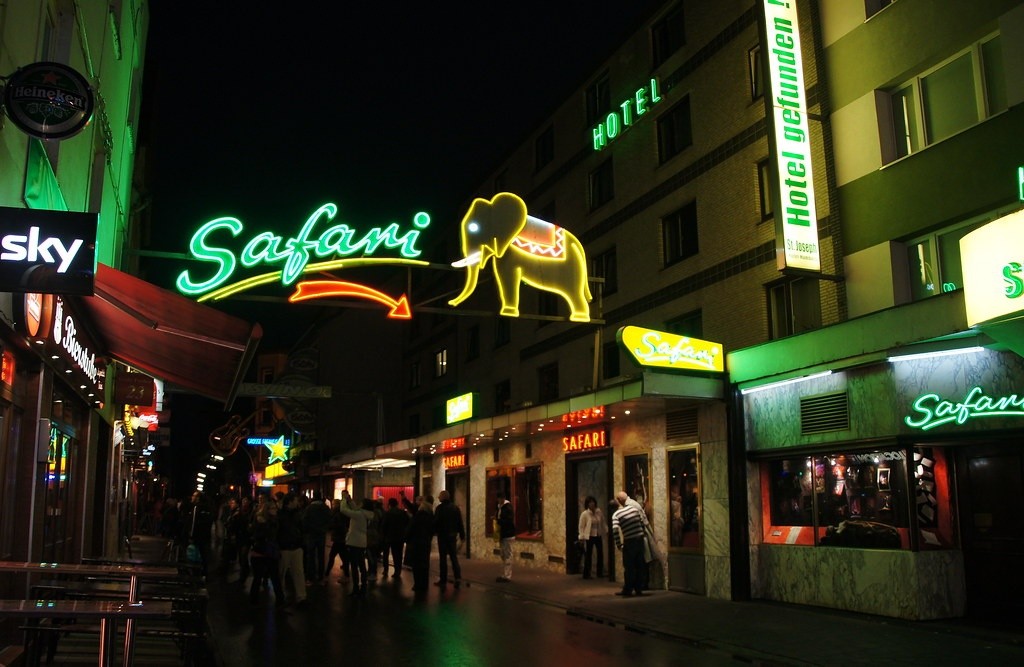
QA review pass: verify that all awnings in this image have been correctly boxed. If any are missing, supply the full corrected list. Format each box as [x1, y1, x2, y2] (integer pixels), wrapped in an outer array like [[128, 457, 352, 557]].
[[82, 263, 261, 413]]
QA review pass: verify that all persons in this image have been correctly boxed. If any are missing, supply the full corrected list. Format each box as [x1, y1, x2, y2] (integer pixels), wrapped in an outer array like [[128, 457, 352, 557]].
[[433, 491, 465, 585], [672, 500, 682, 546], [491, 493, 514, 583], [578, 497, 608, 580], [616, 491, 655, 590], [161, 490, 434, 609], [609, 500, 644, 597]]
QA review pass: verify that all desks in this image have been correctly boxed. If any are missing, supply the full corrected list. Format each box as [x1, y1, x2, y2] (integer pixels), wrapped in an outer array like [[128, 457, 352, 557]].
[[0, 599, 172, 667], [0, 561, 179, 667]]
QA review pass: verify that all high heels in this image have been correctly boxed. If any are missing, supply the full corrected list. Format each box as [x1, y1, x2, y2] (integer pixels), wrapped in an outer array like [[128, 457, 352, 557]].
[[348, 585, 361, 599], [359, 584, 368, 598]]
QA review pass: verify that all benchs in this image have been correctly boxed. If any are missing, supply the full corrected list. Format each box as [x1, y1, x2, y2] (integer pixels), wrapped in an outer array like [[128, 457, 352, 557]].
[[17, 565, 209, 667]]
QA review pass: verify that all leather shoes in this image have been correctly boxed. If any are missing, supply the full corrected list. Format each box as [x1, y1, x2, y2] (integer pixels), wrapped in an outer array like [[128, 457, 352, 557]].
[[616, 591, 632, 596], [434, 582, 447, 586]]
